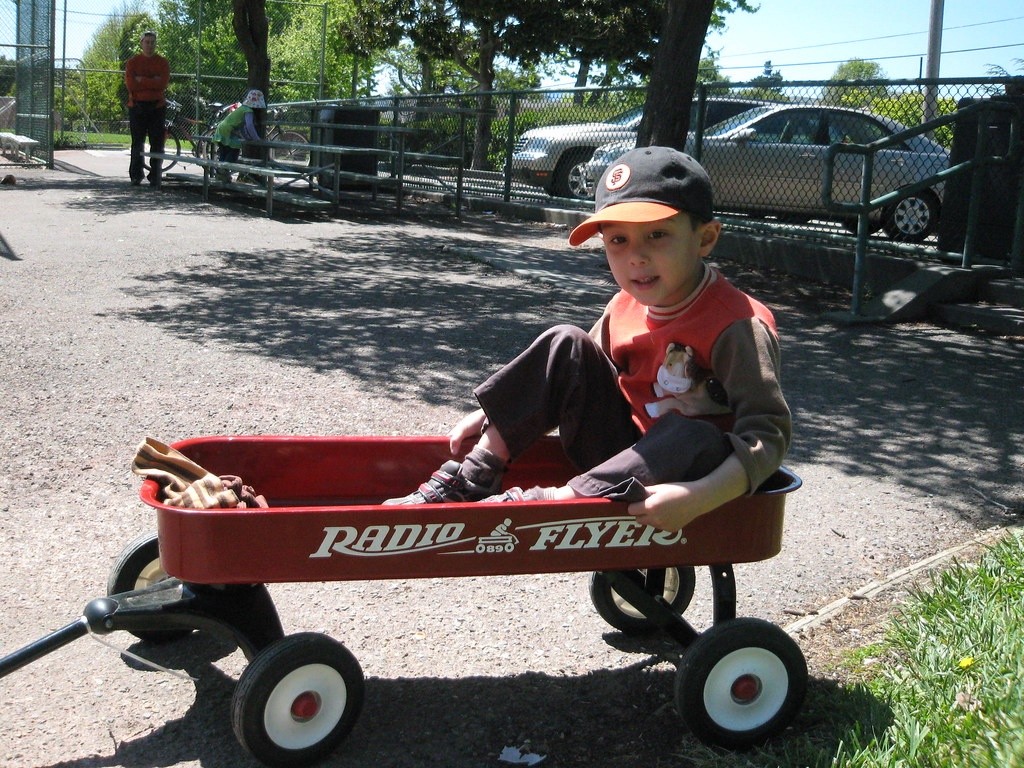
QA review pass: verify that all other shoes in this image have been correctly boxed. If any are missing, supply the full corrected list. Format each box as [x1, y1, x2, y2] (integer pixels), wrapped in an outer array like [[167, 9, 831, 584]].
[[383, 461, 504, 507], [478, 487, 538, 502]]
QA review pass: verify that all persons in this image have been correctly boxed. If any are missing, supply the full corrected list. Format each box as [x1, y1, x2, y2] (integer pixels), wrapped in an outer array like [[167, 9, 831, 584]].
[[210, 89, 267, 183], [125, 32, 171, 189], [383, 147, 793, 536]]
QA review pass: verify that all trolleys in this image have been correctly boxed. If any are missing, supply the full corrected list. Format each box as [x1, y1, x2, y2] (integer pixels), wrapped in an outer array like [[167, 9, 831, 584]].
[[0, 436, 808, 768]]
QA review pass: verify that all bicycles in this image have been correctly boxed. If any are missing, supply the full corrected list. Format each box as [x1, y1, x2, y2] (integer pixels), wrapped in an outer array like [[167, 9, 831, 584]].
[[141, 97, 312, 184]]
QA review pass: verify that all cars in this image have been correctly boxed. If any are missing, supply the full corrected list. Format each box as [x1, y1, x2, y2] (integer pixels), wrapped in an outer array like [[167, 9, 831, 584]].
[[586, 103, 952, 242], [506, 95, 848, 225]]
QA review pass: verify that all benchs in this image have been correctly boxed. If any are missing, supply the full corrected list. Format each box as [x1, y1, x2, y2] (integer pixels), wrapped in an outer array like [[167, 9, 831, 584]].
[[0, 132, 17, 158], [7, 135, 40, 162], [139, 97, 468, 227]]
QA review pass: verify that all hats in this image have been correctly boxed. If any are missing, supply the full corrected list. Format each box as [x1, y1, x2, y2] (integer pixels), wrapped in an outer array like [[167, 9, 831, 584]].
[[244, 90, 266, 108], [568, 146, 712, 247]]
